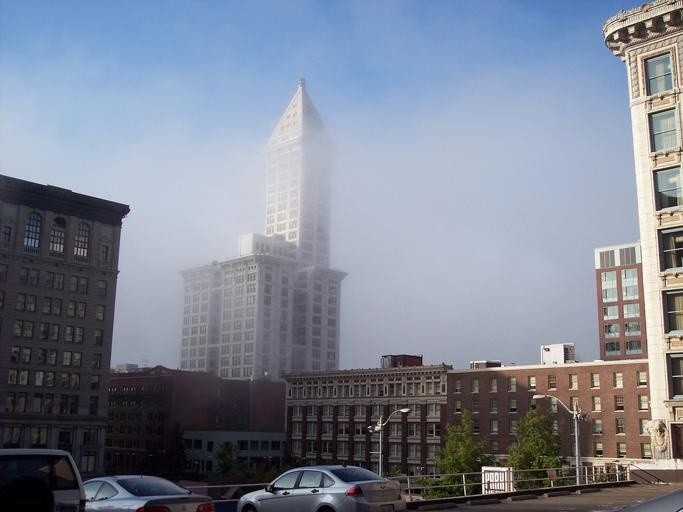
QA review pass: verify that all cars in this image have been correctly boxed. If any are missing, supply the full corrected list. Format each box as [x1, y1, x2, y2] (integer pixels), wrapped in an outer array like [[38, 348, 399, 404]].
[[0, 449, 86, 511], [81, 473, 215, 511], [237, 460, 408, 512]]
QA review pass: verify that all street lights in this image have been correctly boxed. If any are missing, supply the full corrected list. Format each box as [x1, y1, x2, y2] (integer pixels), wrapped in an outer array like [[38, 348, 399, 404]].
[[250, 365, 268, 407], [532, 394, 583, 485], [378, 408, 411, 472]]
